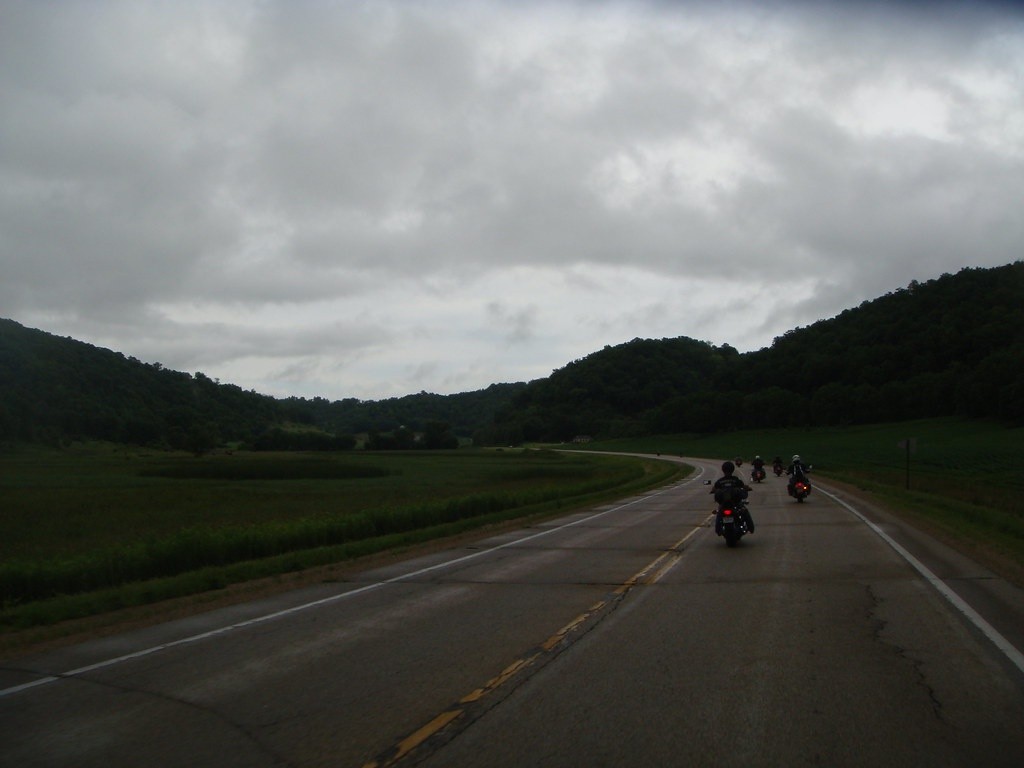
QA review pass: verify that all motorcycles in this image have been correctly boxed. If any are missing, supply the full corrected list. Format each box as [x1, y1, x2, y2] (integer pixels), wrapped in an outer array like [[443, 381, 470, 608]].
[[703, 477, 759, 549], [783, 464, 813, 503], [752, 463, 766, 483], [773, 463, 783, 477]]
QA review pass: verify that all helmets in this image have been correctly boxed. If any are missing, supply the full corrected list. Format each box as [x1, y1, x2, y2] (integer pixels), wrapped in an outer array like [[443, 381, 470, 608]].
[[792, 455, 800, 462], [755, 455, 760, 459]]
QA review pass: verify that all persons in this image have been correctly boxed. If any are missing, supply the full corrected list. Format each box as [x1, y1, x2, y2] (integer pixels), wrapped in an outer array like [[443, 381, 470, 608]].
[[772, 455, 784, 470], [751, 456, 765, 477], [787, 455, 812, 496], [709, 461, 750, 533]]
